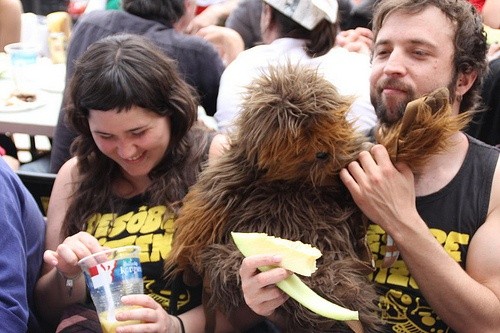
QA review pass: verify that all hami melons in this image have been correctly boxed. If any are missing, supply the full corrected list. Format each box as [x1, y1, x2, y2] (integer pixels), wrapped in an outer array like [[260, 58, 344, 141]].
[[231, 231, 358, 321]]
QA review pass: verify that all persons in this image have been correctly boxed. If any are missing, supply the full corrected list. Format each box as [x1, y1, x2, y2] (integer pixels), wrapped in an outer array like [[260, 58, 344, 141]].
[[34, 32, 234, 333], [238, 0, 500, 333], [0, 155, 48, 333], [0, 0, 500, 174]]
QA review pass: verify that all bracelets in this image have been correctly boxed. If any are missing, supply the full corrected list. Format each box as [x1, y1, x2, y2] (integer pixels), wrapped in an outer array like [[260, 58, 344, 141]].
[[173, 315, 186, 333], [55, 267, 83, 300]]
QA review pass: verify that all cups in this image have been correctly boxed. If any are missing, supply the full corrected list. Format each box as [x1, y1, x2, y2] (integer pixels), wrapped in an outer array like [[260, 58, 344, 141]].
[[77, 246, 146, 333], [5, 42, 45, 97]]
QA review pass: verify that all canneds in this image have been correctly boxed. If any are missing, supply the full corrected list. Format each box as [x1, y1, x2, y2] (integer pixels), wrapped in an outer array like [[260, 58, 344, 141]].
[[47, 11, 72, 63]]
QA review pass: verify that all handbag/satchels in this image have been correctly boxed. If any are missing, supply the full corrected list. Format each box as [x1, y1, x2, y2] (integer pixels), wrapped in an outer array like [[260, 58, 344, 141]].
[[55, 304, 103, 333]]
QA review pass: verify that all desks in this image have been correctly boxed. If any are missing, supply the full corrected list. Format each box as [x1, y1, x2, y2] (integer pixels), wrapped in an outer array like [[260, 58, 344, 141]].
[[0, 52, 66, 138]]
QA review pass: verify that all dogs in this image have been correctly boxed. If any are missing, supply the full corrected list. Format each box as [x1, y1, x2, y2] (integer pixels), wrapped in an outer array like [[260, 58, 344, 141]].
[[162, 53, 488, 330]]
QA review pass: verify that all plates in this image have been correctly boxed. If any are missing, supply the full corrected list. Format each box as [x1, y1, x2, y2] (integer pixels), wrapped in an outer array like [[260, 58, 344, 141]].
[[0, 92, 47, 112]]
